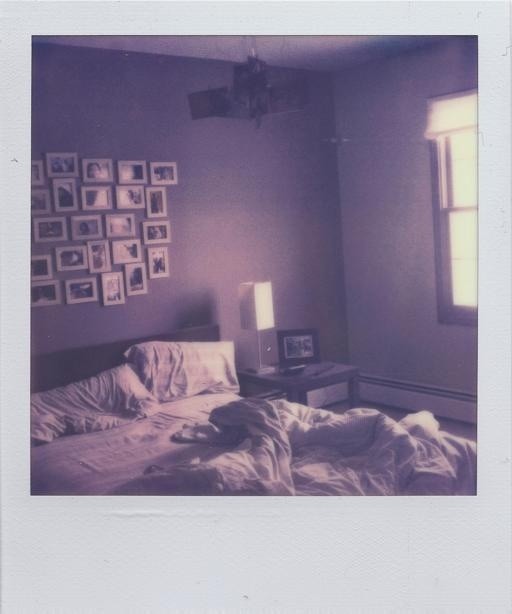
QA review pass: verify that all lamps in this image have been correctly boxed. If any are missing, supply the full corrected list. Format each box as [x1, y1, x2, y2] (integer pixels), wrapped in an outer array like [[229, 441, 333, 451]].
[[186, 39, 307, 128], [237, 281, 275, 374]]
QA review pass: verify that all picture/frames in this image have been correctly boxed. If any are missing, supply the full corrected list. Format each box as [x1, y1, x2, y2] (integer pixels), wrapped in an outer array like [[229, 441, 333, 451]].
[[31, 152, 178, 307], [278, 328, 320, 366]]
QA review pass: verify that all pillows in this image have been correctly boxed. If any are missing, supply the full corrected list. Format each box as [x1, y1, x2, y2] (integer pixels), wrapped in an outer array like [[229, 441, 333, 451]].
[[125, 340, 241, 402], [31, 363, 163, 445]]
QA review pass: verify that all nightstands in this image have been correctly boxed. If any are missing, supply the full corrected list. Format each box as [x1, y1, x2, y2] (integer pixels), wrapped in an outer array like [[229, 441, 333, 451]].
[[238, 359, 358, 413]]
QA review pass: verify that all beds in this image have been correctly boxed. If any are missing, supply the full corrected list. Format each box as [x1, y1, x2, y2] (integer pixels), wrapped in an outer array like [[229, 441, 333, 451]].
[[30, 324, 477, 495]]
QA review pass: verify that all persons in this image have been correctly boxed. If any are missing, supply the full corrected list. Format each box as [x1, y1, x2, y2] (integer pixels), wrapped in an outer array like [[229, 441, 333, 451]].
[[87, 163, 102, 178], [127, 190, 137, 207], [80, 223, 89, 235], [152, 193, 158, 213], [52, 160, 71, 172]]
